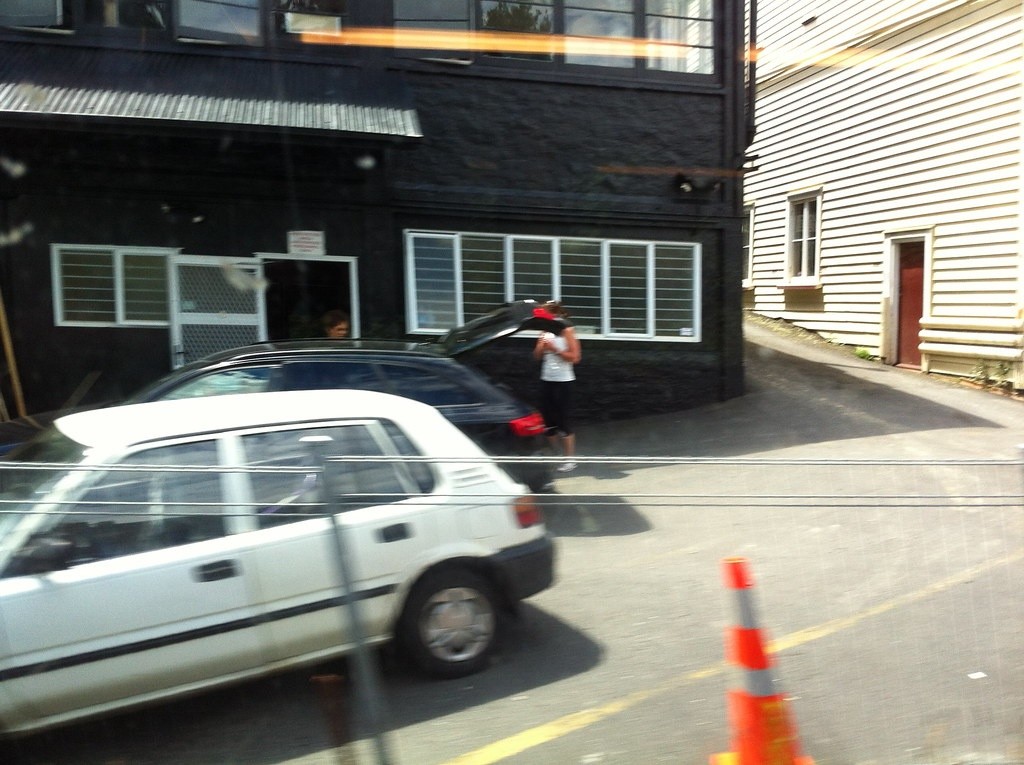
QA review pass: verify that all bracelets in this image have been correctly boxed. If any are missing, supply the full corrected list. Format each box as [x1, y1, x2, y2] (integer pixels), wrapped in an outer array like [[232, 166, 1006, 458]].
[[555, 349, 563, 356]]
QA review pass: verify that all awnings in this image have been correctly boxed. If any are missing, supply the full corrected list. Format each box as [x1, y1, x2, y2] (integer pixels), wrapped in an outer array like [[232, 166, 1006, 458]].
[[1, 81, 425, 139]]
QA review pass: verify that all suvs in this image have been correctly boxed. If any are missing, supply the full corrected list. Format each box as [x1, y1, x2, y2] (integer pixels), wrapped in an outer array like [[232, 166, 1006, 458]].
[[0, 390, 552, 748], [0, 298, 563, 505]]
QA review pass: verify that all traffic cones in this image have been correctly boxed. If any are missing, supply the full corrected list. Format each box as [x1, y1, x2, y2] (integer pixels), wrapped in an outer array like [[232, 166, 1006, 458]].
[[708, 557, 818, 765]]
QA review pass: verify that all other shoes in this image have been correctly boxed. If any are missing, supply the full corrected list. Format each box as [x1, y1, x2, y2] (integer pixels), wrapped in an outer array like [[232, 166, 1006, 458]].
[[557, 463, 577, 472]]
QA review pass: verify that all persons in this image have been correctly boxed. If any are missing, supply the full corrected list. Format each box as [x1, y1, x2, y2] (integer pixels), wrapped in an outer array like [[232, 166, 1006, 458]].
[[322, 310, 350, 338], [532, 300, 580, 473]]
